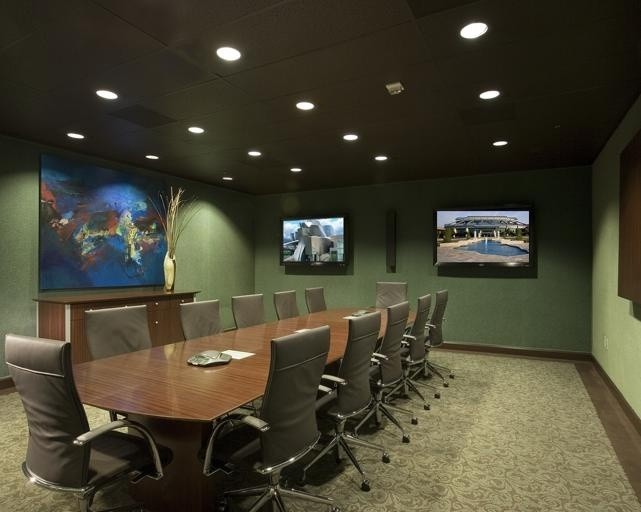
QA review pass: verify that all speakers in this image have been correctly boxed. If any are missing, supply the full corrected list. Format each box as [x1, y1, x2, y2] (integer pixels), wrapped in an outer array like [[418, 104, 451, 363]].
[[385, 208, 396, 273]]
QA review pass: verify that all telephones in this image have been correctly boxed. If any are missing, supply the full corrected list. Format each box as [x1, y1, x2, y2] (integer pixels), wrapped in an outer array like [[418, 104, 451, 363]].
[[186, 349, 232, 367]]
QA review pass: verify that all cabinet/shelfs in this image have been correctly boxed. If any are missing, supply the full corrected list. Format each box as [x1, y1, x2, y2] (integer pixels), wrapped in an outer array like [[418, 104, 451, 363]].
[[30, 289, 203, 367]]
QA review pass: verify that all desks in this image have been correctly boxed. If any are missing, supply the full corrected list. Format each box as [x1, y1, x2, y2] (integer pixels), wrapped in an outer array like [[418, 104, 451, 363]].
[[72, 306, 418, 512]]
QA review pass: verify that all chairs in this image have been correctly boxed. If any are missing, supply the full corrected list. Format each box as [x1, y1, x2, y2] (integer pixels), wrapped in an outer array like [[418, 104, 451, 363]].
[[230, 292, 267, 330], [272, 288, 302, 321], [80, 303, 176, 446], [179, 297, 223, 342], [201, 322, 344, 512], [302, 285, 328, 315], [369, 280, 457, 427], [295, 309, 392, 494], [0, 331, 174, 512]]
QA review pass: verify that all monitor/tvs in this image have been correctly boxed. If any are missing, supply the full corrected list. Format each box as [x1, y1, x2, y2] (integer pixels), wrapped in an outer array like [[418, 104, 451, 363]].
[[279, 212, 348, 266], [432, 204, 536, 267]]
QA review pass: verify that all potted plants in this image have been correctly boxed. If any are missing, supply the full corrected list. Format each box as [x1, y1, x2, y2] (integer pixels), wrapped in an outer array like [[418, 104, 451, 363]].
[[148, 185, 204, 290]]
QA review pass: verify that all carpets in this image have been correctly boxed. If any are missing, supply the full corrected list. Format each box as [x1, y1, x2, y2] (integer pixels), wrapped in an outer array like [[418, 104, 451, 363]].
[[0, 344, 640, 512]]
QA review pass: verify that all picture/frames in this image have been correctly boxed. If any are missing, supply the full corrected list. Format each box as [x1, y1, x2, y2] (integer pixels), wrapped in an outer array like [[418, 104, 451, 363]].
[[37, 152, 168, 291]]
